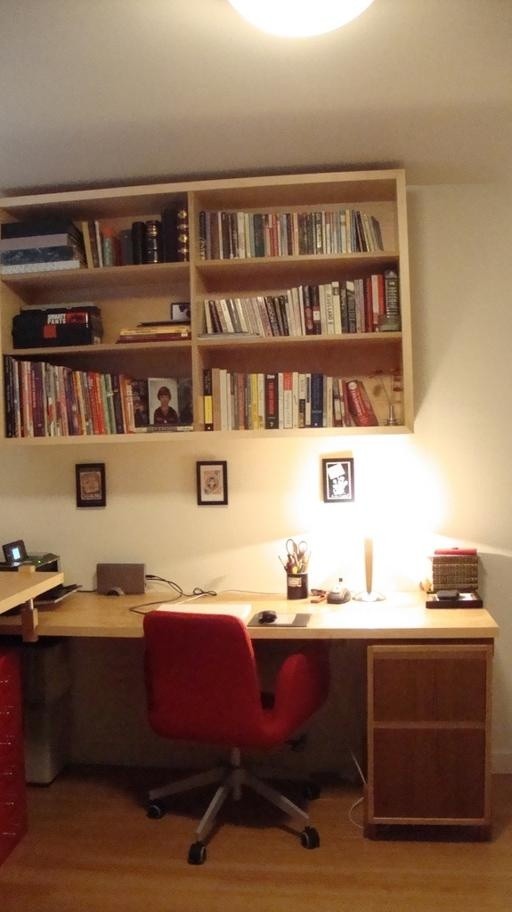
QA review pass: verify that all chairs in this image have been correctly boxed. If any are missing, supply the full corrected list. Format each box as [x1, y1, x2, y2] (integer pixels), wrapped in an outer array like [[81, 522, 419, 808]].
[[141, 611, 328, 865]]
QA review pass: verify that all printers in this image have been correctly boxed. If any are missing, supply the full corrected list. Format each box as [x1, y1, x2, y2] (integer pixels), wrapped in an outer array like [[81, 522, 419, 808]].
[[0, 540, 83, 605]]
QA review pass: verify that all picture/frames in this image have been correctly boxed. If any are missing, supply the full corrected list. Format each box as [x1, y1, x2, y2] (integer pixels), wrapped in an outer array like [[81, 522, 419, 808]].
[[322, 458, 355, 503], [75, 462, 106, 507], [197, 460, 228, 505]]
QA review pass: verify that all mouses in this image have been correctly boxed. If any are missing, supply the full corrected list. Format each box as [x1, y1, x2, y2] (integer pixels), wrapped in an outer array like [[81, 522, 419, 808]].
[[261, 610, 277, 622]]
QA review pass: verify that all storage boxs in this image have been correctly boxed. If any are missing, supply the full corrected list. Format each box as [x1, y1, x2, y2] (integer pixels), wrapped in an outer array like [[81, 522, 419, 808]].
[[11, 306, 103, 349]]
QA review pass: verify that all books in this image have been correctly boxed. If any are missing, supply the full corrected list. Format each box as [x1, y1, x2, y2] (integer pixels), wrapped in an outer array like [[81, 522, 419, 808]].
[[116, 318, 191, 343], [426, 554, 480, 592], [204, 369, 380, 431], [6, 356, 149, 438], [199, 211, 384, 259], [1, 199, 189, 275], [197, 269, 399, 339], [12, 301, 103, 350]]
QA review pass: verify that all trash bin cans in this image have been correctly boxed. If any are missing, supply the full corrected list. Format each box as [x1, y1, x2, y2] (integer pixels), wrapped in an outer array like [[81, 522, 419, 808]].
[[19, 637, 74, 784]]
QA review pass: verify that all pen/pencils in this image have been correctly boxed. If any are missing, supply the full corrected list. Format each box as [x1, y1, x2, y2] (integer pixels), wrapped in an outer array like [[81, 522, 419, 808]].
[[279, 552, 311, 573]]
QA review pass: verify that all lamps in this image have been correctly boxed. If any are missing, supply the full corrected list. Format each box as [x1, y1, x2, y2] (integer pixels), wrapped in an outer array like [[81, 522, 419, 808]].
[[232, 0, 371, 35]]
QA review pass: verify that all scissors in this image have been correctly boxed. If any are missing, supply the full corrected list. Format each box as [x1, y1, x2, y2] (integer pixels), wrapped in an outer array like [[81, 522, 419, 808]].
[[286, 538, 308, 556]]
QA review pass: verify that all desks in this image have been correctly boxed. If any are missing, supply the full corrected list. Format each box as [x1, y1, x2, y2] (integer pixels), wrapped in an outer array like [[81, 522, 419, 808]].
[[0, 594, 500, 840], [0, 571, 64, 867]]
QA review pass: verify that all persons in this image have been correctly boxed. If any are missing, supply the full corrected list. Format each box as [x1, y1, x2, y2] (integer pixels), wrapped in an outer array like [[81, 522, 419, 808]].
[[207, 476, 217, 490], [153, 386, 179, 423]]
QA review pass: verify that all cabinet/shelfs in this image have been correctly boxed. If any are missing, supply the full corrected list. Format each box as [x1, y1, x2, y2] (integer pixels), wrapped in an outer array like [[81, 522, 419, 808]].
[[0, 645, 29, 866], [1, 169, 415, 444]]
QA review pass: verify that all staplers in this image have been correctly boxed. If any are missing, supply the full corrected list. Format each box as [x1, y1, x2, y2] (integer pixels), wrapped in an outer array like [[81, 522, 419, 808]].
[[311, 589, 328, 604]]
